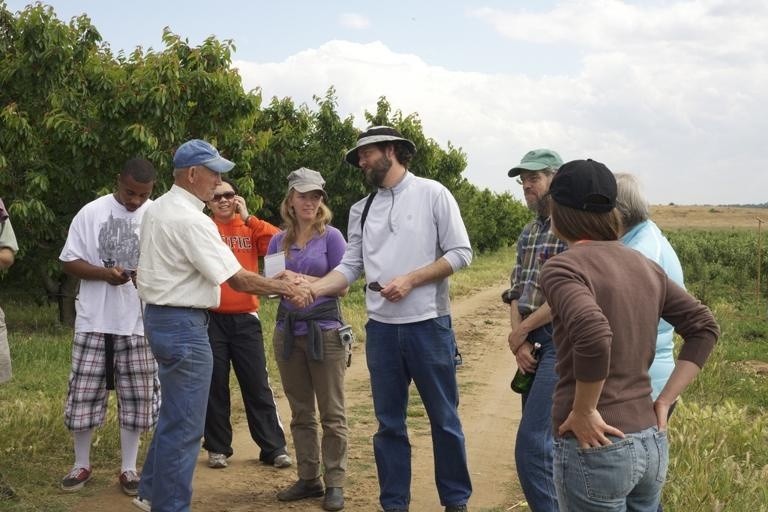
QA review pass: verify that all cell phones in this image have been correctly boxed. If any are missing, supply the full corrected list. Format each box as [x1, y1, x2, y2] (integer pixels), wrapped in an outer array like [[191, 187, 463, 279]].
[[122, 267, 136, 278]]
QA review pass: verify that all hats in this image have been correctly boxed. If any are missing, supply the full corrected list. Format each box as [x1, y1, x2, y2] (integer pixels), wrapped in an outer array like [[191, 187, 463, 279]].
[[541, 160, 618, 212], [345, 126, 417, 167], [287, 167, 328, 201], [172, 139, 236, 174], [508, 149, 562, 175]]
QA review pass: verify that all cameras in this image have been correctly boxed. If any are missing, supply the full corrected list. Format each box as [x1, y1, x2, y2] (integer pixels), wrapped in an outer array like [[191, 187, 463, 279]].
[[338, 324, 353, 346]]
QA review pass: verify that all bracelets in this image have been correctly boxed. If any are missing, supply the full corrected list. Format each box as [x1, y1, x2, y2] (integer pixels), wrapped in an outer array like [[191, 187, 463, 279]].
[[244, 215, 252, 226]]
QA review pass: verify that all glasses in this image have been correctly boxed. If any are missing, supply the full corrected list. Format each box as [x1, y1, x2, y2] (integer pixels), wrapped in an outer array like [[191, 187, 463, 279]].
[[516, 176, 539, 184], [212, 192, 235, 200]]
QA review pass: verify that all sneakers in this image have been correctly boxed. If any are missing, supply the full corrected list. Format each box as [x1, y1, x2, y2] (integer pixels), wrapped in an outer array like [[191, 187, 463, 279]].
[[444, 503, 467, 512], [132, 497, 152, 512], [120, 470, 141, 497], [208, 451, 227, 468], [324, 487, 344, 510], [61, 467, 93, 491], [273, 454, 292, 467], [277, 479, 323, 501]]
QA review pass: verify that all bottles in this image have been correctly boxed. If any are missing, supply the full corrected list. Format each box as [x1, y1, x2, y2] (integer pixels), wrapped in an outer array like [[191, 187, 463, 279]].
[[511, 342, 542, 394]]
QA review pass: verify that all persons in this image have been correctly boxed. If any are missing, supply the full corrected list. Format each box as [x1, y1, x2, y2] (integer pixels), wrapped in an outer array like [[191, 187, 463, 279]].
[[263, 167, 353, 512], [604, 171, 687, 426], [503, 149, 564, 511], [131, 138, 322, 511], [282, 126, 474, 512], [58, 157, 155, 498], [535, 159, 720, 511], [201, 178, 292, 469], [1, 196, 21, 387]]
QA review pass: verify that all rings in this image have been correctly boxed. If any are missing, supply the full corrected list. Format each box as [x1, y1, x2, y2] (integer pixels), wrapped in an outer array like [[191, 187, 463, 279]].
[[391, 296, 396, 299]]
